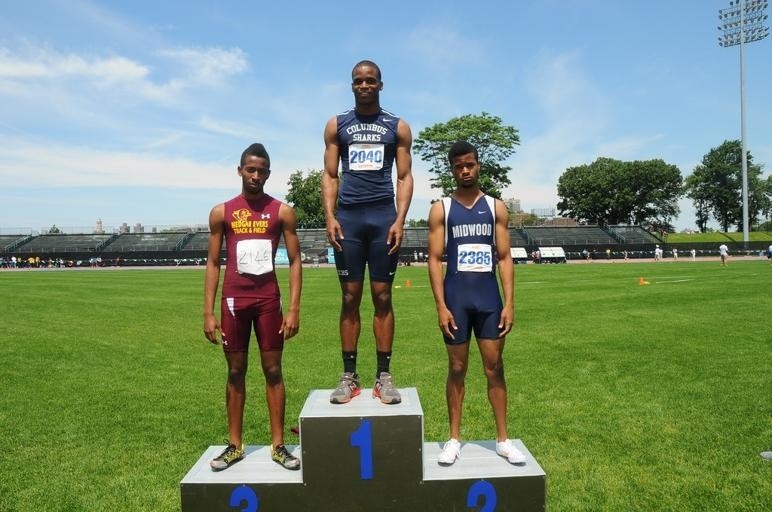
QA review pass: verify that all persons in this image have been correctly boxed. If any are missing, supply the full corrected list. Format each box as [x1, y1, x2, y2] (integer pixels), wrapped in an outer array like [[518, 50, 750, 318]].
[[203, 142, 304, 470], [672, 248, 677, 260], [320, 59, 415, 404], [530, 248, 541, 264], [1, 255, 107, 270], [427, 141, 527, 465], [690, 248, 696, 260], [115, 256, 124, 265], [718, 242, 728, 267], [622, 249, 628, 262], [653, 245, 664, 262], [766, 243, 771, 259], [576, 246, 619, 262]]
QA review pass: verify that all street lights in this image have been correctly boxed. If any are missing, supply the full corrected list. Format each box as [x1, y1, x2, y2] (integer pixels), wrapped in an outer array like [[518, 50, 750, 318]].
[[717, 0, 770, 242]]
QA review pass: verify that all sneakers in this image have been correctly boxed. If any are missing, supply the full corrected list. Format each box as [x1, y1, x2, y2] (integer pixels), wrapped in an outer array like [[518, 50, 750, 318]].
[[437, 437, 462, 465], [209, 438, 244, 470], [496, 438, 528, 465], [270, 443, 301, 471], [371, 371, 402, 405], [328, 370, 362, 405]]
